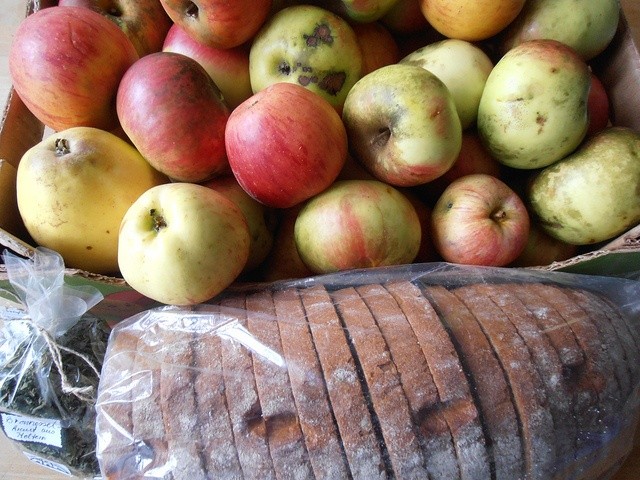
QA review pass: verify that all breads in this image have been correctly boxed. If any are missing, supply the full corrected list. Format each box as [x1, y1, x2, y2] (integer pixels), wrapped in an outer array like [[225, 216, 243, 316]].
[[94, 280, 640, 480]]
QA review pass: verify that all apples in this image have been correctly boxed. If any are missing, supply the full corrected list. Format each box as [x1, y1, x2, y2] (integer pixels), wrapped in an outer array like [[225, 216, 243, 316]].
[[8, 2, 640, 307]]
